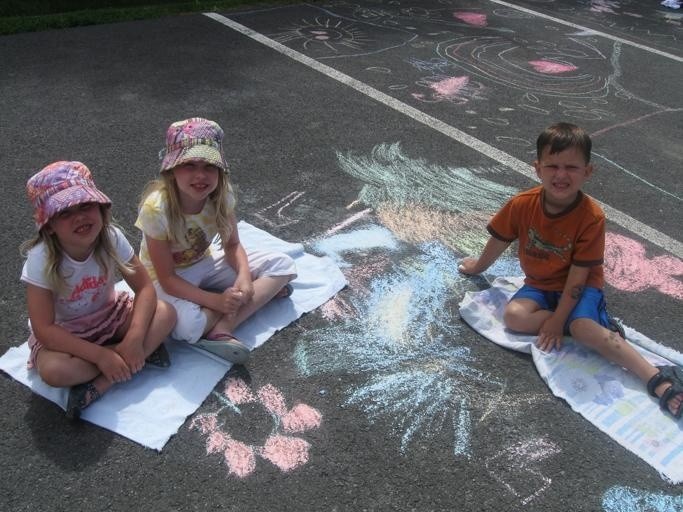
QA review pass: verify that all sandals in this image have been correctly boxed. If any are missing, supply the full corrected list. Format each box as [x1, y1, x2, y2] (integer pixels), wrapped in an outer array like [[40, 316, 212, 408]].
[[647, 365, 683, 418], [196, 334, 250, 364]]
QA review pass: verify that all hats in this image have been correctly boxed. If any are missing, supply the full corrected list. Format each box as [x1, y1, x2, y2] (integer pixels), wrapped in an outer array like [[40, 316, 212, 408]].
[[26, 160, 112, 232], [158, 117, 224, 173]]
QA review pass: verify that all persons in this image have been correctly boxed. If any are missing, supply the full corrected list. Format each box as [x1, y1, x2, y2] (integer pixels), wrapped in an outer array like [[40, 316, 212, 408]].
[[18, 159, 180, 422], [456, 120, 682, 424], [133, 116, 299, 368]]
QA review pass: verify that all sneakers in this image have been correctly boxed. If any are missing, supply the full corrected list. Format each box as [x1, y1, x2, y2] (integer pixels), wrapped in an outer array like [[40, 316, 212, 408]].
[[146, 344, 170, 368], [66, 381, 100, 419]]
